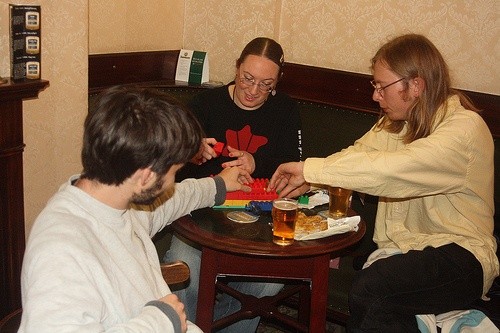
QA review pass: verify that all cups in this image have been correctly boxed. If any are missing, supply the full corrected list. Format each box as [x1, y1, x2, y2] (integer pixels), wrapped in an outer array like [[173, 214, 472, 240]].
[[329, 186, 350, 220], [271, 198, 299, 246]]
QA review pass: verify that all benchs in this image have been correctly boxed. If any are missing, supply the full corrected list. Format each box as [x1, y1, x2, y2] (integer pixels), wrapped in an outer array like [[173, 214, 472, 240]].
[[415, 307, 500, 333], [87, 52, 500, 333]]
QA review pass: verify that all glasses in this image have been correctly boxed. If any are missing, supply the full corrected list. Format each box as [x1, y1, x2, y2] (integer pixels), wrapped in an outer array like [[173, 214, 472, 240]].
[[238, 65, 278, 92], [369, 77, 406, 93]]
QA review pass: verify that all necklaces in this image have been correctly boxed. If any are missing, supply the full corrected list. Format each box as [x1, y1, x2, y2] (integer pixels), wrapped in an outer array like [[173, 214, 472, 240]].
[[233, 85, 267, 152]]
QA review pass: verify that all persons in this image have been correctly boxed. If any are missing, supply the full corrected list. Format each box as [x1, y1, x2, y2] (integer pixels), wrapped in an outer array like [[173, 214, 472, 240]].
[[16, 84, 255, 333], [162, 37, 303, 333], [267, 34, 500, 333]]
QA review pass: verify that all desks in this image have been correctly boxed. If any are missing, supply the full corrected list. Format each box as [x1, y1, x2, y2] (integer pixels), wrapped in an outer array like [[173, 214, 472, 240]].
[[166, 201, 367, 333]]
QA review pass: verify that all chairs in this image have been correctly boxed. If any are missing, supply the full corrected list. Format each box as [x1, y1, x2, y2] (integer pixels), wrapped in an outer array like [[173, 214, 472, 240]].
[[0, 262, 190, 333]]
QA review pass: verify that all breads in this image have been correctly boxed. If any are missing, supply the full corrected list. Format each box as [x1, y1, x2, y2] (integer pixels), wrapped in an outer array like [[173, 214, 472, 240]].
[[295, 212, 328, 234]]
